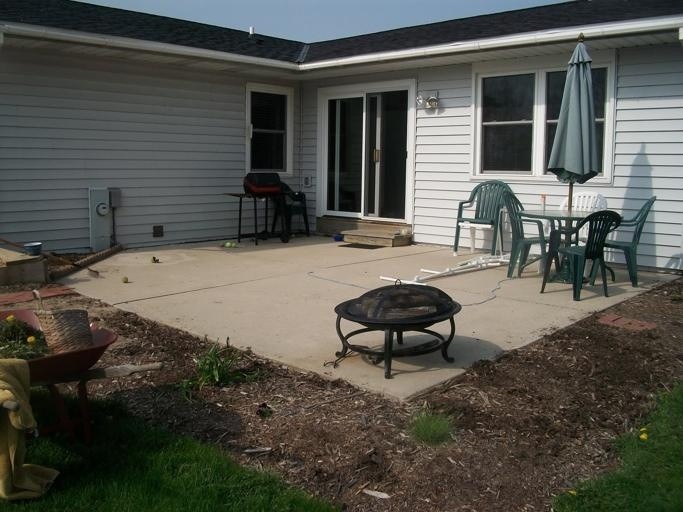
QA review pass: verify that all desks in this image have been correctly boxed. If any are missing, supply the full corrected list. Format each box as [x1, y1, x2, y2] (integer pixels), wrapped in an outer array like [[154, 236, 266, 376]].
[[223, 191, 270, 245]]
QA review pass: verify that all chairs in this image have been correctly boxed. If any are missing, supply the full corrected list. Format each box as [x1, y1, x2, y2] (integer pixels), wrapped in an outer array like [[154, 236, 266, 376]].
[[454, 180, 656, 301], [270, 182, 311, 237]]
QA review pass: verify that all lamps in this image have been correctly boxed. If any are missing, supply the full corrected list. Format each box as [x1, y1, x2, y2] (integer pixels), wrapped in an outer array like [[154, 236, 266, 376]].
[[415, 91, 439, 109]]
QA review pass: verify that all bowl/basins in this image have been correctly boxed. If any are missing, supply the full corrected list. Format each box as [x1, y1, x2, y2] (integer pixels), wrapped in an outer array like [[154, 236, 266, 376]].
[[24, 242, 42, 256]]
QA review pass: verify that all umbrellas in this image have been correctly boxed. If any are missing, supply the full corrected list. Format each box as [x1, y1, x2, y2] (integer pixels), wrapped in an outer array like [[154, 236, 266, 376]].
[[543, 30, 601, 248]]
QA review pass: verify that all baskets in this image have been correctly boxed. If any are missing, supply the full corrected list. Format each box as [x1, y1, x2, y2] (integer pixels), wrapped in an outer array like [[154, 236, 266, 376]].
[[30, 288, 95, 353]]
